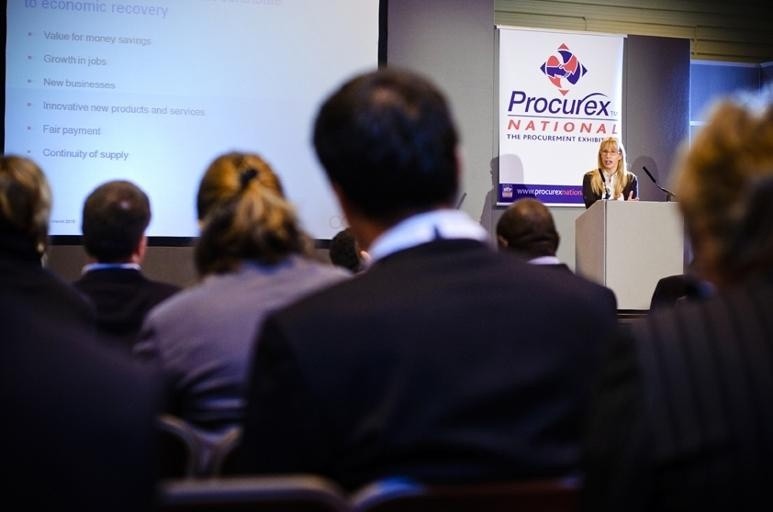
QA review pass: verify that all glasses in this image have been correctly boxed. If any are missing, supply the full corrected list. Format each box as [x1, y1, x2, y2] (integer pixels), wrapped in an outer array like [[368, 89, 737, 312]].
[[599, 150, 621, 156]]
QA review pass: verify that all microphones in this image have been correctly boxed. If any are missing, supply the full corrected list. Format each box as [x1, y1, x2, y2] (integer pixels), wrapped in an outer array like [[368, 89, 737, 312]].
[[643, 166, 678, 202]]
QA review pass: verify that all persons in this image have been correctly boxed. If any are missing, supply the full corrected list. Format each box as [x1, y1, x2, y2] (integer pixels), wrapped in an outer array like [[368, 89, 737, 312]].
[[584, 86, 770, 509], [3, 153, 164, 504], [582, 137, 639, 211], [328, 225, 372, 276], [68, 180, 180, 363], [131, 150, 356, 435], [241, 70, 615, 494], [495, 197, 572, 274]]
